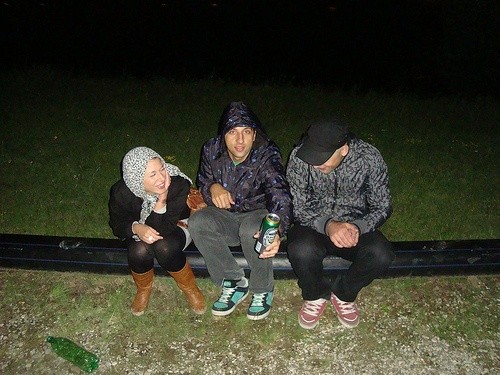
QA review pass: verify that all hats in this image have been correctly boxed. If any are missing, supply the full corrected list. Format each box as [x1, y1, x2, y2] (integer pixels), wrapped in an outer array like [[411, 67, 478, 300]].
[[295, 116, 349, 166]]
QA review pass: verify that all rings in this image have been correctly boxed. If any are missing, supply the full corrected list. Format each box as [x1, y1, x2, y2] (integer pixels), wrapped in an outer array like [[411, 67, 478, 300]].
[[148, 235, 153, 241]]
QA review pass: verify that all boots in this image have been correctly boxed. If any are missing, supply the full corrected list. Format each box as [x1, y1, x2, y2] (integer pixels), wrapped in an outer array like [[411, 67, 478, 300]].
[[130, 268, 155, 316], [167, 258, 207, 315]]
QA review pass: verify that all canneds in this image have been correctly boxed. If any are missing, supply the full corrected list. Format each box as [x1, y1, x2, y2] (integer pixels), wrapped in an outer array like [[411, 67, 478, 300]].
[[254, 213, 280, 254]]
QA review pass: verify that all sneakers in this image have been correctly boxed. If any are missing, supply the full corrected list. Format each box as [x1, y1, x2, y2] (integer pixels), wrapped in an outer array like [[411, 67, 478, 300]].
[[298, 298, 328, 330], [246, 287, 275, 320], [330, 291, 360, 329], [211, 275, 249, 316]]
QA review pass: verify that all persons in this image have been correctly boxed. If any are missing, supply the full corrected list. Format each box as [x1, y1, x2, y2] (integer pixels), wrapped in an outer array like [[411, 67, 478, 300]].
[[187, 102, 292, 320], [285, 118, 392, 329], [109, 146, 207, 316]]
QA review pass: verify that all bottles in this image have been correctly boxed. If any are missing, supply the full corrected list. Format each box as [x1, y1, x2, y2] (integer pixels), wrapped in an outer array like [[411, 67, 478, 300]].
[[47, 336, 100, 374]]
